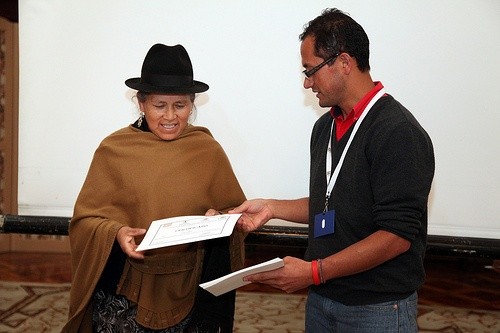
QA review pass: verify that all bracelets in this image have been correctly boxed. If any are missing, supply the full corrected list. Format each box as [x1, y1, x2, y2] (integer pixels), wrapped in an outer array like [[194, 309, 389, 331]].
[[311, 259, 324, 285]]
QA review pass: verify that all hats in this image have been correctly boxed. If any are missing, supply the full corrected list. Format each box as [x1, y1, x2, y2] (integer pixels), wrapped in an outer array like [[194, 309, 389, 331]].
[[124, 42, 209, 94]]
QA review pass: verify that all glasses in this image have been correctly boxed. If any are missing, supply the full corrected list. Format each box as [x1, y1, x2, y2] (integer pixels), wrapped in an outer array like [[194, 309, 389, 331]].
[[301, 50, 355, 79]]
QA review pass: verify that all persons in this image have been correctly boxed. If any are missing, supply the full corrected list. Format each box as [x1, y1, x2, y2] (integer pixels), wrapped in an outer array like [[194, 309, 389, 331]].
[[60, 44, 248, 333], [229, 7, 436, 333]]
